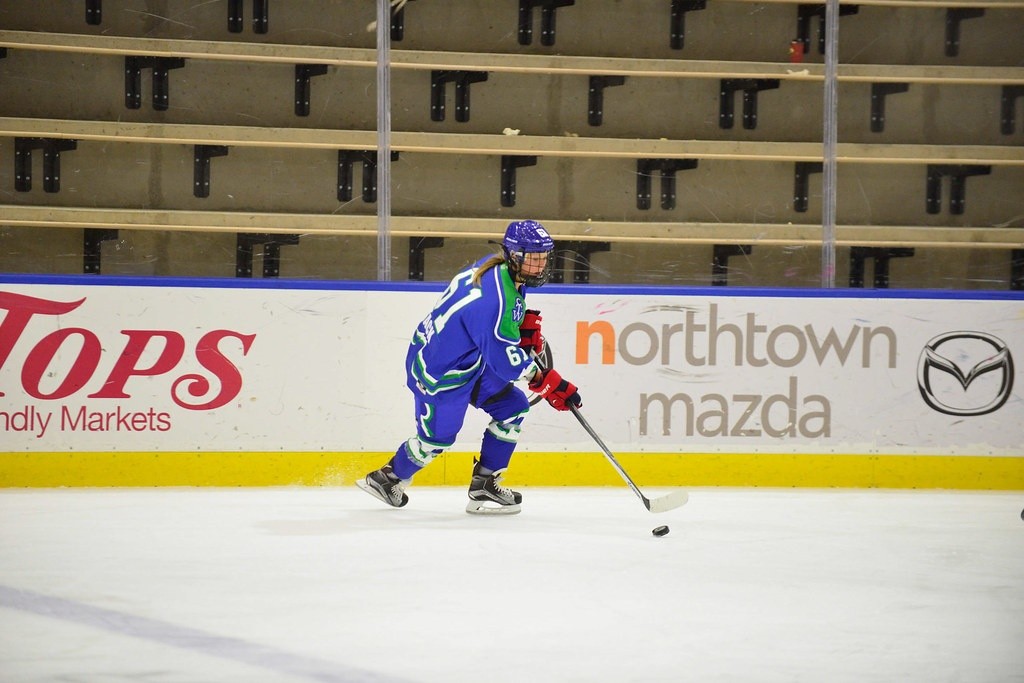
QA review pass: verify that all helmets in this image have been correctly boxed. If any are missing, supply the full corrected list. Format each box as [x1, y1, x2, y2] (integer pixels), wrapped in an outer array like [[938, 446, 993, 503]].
[[502, 219, 554, 287]]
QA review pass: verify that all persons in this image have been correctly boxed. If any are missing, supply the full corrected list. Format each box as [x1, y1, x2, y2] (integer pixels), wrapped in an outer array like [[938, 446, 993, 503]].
[[355, 220, 581, 515]]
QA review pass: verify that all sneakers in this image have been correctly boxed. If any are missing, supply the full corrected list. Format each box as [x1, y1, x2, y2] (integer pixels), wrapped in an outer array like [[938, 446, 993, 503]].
[[354, 457, 409, 507], [465, 456, 522, 513]]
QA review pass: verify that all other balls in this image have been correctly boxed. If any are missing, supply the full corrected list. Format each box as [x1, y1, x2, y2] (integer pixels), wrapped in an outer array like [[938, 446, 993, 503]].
[[652, 526, 670, 537]]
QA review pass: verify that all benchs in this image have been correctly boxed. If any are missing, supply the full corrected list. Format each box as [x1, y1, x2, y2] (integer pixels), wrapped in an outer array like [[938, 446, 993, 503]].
[[0, 1, 1024, 293]]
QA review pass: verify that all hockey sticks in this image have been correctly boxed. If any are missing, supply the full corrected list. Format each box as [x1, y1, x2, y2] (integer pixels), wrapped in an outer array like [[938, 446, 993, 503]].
[[529, 348, 688, 514]]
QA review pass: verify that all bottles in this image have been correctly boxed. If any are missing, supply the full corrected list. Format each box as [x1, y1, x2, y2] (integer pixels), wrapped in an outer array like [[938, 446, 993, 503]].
[[792, 39, 804, 63]]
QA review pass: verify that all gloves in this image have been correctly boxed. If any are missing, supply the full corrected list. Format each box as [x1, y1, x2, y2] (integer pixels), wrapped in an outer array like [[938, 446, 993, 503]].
[[528, 368, 583, 411], [520, 309, 544, 355]]
